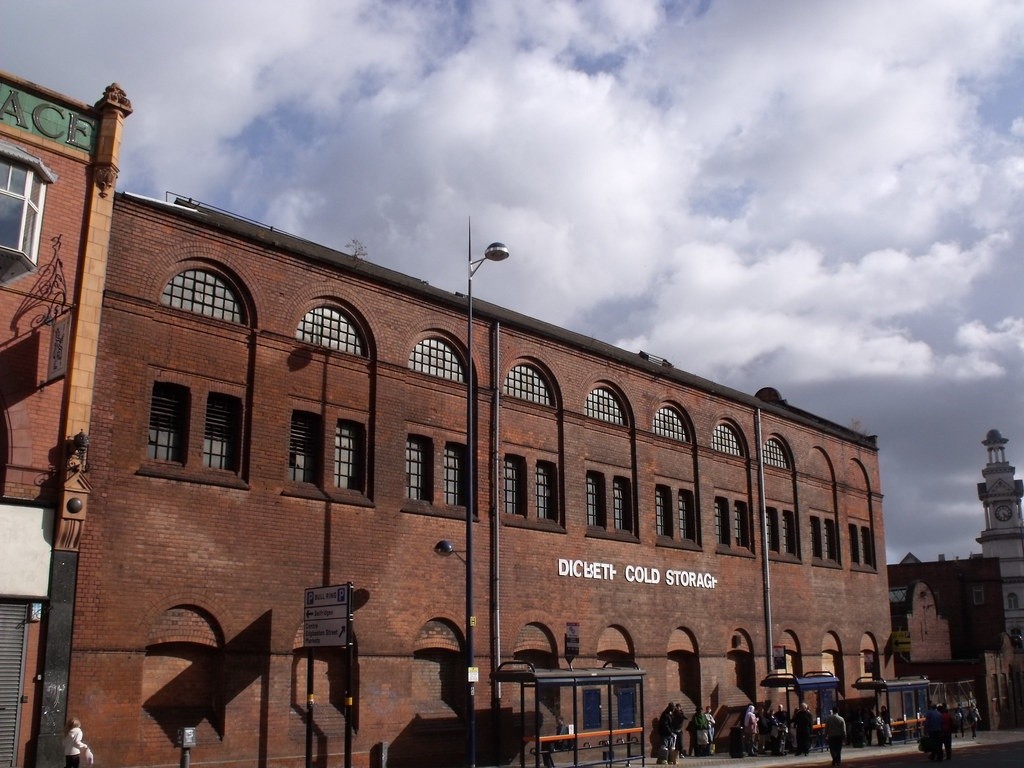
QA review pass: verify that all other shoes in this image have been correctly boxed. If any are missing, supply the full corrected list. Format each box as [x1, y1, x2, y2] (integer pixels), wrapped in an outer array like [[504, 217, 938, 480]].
[[795, 753, 801, 756], [748, 753, 757, 757], [837, 764, 840, 766], [805, 752, 808, 756], [832, 763, 835, 765]]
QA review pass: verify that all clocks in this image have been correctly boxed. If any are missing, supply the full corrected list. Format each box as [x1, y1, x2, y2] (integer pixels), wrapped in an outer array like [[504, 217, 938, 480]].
[[995, 505, 1013, 522]]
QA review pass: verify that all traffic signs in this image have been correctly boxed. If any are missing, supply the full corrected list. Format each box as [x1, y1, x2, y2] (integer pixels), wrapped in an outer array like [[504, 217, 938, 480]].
[[303, 618, 352, 648], [303, 603, 350, 619]]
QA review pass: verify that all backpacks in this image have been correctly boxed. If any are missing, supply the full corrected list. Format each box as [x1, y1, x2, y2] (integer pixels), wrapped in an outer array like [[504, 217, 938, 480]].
[[954, 712, 962, 722], [967, 708, 978, 724]]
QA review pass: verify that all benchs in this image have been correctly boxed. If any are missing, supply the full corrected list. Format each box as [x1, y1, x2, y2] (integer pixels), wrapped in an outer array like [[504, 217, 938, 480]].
[[892, 725, 924, 733], [530, 736, 639, 754]]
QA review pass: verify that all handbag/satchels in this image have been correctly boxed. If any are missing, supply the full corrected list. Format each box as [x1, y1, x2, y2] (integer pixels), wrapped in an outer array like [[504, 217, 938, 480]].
[[696, 729, 709, 745], [883, 724, 892, 738], [669, 749, 679, 765], [85, 746, 95, 766], [770, 725, 779, 738], [919, 736, 932, 753]]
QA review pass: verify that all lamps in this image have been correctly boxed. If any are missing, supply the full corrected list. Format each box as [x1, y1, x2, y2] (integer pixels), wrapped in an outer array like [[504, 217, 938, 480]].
[[65, 429, 91, 473]]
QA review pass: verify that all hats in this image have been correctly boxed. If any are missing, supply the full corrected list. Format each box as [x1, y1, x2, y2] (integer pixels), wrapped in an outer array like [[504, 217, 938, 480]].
[[668, 701, 677, 709]]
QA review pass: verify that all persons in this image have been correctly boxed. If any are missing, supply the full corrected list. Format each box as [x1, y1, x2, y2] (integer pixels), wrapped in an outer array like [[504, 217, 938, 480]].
[[659, 702, 981, 768], [555, 717, 567, 746], [63, 718, 87, 768]]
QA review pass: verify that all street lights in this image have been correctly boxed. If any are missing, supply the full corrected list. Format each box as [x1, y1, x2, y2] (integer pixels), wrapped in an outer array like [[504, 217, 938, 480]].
[[434, 214, 510, 768]]
